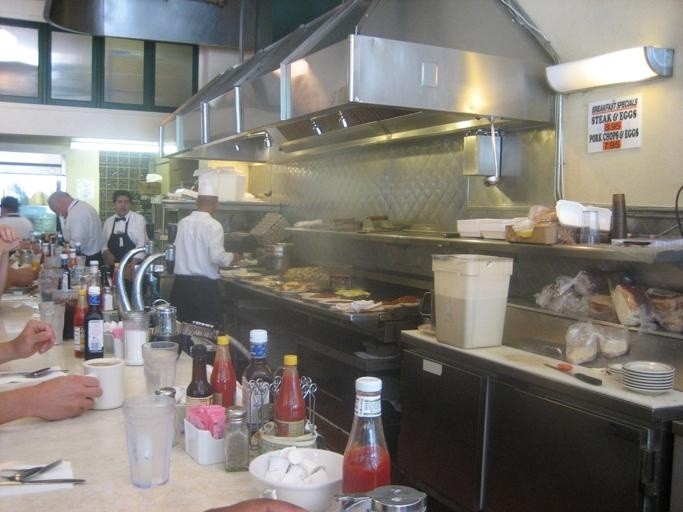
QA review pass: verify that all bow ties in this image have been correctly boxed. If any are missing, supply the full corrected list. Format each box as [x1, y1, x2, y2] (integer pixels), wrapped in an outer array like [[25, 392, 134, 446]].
[[115, 217, 126, 221]]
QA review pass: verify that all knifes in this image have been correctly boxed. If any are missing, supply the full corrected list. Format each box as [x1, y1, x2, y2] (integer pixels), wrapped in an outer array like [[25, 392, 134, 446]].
[[0, 478, 85, 486]]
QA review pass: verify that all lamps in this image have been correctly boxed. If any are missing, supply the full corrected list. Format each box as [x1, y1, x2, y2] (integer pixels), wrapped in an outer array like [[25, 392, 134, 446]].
[[545, 47, 673, 94]]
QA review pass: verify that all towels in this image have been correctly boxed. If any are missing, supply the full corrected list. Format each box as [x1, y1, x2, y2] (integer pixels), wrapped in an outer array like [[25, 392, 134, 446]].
[[3, 321, 29, 332], [0, 366, 66, 384], [335, 298, 383, 314], [0, 460, 73, 495]]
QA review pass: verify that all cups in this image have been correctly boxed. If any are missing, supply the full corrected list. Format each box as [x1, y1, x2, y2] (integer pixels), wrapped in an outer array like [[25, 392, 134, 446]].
[[53, 293, 77, 340], [579, 210, 601, 245], [611, 193, 626, 240], [38, 301, 66, 345], [77, 340, 178, 489]]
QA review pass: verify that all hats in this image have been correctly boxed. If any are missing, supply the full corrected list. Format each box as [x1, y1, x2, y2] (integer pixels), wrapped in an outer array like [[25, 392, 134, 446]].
[[197, 170, 220, 196]]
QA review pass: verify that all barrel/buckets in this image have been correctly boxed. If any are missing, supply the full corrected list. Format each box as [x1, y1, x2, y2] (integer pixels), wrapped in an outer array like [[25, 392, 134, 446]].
[[432, 254, 515, 350]]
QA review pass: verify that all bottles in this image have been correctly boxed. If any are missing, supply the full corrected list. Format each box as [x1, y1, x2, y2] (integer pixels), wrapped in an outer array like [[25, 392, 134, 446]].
[[72, 288, 115, 358], [28, 229, 121, 290], [113, 304, 182, 366], [341, 376, 391, 497], [185, 329, 308, 473]]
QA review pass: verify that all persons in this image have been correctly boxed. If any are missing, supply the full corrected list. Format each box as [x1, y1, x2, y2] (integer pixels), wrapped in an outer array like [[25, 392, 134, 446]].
[[172, 169, 240, 330], [0, 196, 33, 237], [48, 192, 105, 270], [102, 190, 149, 303], [0, 225, 30, 294], [0, 320, 103, 423]]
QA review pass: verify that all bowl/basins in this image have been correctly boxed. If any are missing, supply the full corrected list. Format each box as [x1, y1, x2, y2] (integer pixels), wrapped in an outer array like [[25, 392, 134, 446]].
[[250, 447, 344, 511]]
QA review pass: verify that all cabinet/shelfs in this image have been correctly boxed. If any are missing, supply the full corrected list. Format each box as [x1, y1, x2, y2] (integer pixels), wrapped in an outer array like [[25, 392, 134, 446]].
[[151, 199, 280, 266], [396, 349, 683, 511]]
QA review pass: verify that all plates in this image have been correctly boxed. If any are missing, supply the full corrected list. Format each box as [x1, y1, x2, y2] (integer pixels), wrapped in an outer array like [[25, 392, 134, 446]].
[[237, 265, 373, 308], [376, 292, 421, 313], [620, 359, 675, 396], [606, 362, 623, 377]]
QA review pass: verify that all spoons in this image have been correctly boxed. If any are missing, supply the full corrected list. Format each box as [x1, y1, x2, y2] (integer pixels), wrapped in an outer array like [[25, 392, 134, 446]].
[[0, 467, 42, 482], [0, 367, 51, 376]]
